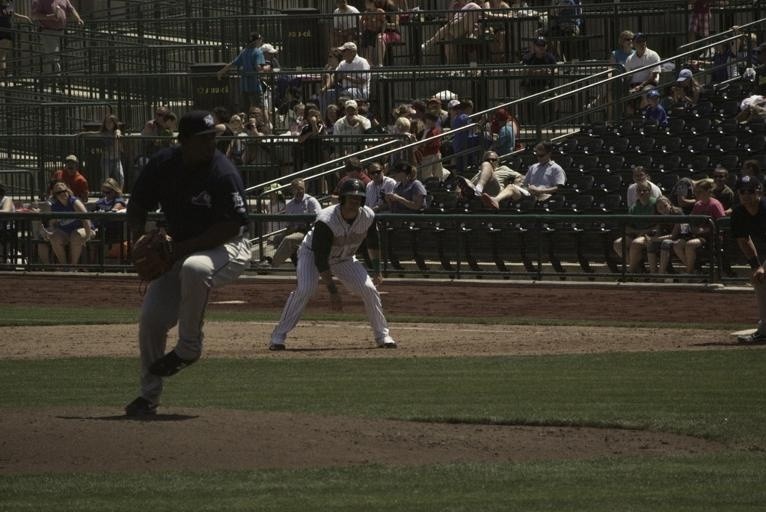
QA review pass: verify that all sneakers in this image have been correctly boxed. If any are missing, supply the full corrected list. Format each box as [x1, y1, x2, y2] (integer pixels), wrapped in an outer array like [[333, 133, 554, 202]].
[[738, 331, 765, 344], [380, 342, 396, 348], [148, 348, 201, 378], [270, 343, 285, 350], [124, 396, 158, 418]]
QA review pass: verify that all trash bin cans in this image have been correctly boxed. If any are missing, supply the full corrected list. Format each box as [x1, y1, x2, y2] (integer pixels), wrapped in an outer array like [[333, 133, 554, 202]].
[[190, 62, 233, 121], [282, 9, 317, 68]]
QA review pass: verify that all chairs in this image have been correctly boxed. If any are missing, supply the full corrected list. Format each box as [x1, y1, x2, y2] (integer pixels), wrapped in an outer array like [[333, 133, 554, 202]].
[[0, 1, 766, 268]]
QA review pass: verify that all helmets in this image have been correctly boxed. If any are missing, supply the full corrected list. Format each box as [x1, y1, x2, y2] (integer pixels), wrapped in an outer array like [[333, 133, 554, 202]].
[[340, 178, 367, 207]]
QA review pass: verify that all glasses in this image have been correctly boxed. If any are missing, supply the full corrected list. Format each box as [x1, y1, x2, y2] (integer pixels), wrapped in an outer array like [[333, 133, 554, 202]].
[[55, 189, 67, 195], [369, 169, 382, 175]]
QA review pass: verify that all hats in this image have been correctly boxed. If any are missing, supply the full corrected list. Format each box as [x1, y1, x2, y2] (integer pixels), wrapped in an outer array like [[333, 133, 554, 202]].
[[247, 31, 463, 131], [735, 174, 762, 190], [535, 36, 546, 47], [677, 68, 693, 82], [178, 110, 221, 138], [65, 154, 80, 162], [647, 90, 660, 98], [633, 33, 647, 39]]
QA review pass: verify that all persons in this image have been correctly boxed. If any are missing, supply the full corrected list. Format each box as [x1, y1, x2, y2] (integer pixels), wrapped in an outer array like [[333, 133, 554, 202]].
[[268, 178, 397, 349], [124, 110, 253, 417], [0, 2, 766, 283], [731, 176, 766, 344]]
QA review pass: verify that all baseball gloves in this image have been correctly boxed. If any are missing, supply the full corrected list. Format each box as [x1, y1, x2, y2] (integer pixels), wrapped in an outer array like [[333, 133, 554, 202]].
[[132, 233, 174, 281]]
